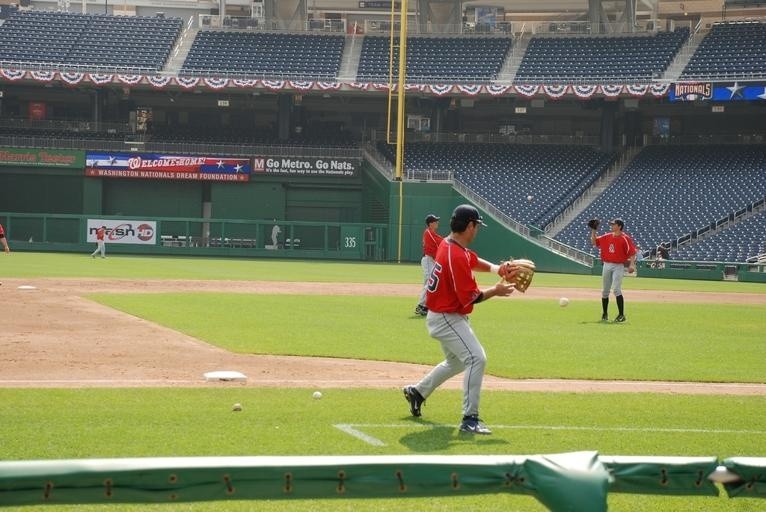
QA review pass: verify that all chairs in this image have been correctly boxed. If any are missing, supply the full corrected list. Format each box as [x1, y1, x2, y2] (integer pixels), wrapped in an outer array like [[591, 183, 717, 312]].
[[0, 10, 766, 264]]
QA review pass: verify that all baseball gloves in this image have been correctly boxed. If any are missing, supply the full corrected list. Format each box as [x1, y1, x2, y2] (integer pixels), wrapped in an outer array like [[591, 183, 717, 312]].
[[499, 259, 535, 293], [589, 219, 600, 229]]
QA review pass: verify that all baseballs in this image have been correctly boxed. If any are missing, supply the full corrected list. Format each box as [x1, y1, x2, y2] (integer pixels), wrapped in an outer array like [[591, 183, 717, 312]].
[[560, 298, 569, 307], [312, 391, 322, 398], [528, 196, 532, 200], [233, 403, 241, 411]]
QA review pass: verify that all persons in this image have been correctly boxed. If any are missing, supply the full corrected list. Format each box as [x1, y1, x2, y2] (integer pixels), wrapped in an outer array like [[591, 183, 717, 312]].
[[657, 243, 670, 259], [403, 205, 516, 434], [0, 223, 10, 285], [591, 217, 638, 322], [634, 245, 644, 261], [415, 214, 445, 316], [91, 226, 108, 260]]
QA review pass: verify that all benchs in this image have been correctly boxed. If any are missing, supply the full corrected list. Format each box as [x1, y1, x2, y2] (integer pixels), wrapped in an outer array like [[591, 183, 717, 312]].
[[285, 239, 300, 248], [160, 236, 258, 248]]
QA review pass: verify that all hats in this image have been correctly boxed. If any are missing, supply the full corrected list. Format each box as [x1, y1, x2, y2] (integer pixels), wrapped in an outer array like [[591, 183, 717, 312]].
[[611, 219, 624, 226], [425, 214, 440, 223], [450, 204, 488, 228]]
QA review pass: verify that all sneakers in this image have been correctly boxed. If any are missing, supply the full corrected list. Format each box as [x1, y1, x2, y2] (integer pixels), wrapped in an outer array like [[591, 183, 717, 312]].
[[614, 314, 625, 322], [415, 304, 422, 314], [602, 312, 608, 320], [402, 384, 425, 418], [420, 306, 427, 315], [460, 416, 493, 436]]
